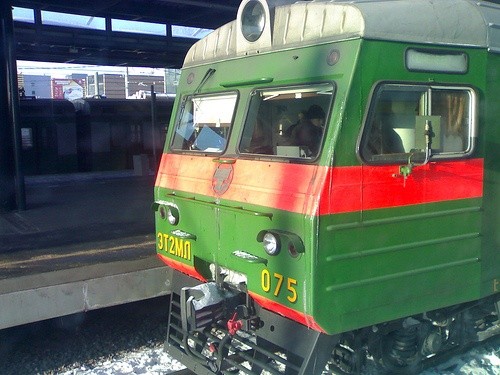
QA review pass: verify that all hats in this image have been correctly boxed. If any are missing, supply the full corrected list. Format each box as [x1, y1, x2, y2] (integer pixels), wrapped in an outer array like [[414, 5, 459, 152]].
[[304, 105, 327, 119]]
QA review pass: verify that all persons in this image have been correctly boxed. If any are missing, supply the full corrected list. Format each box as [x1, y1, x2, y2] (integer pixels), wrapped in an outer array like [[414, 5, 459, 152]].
[[366, 96, 406, 153], [292, 102, 326, 154], [285, 109, 306, 137]]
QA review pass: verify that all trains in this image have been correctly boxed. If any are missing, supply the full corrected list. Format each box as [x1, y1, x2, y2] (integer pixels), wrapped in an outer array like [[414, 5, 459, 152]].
[[151, 0, 500, 375]]
